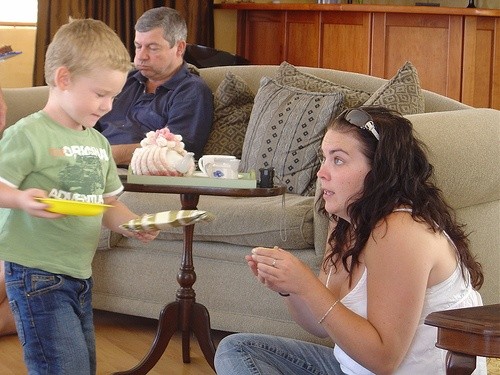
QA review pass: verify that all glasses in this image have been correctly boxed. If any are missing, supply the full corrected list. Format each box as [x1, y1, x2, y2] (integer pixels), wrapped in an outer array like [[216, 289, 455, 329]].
[[334, 107, 382, 141]]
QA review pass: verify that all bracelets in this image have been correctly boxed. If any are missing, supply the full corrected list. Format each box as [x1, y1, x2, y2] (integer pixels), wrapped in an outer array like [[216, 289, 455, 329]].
[[318, 299, 339, 324]]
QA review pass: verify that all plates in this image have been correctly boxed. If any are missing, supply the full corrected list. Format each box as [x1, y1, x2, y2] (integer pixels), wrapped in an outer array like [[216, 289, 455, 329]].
[[34, 197, 115, 217]]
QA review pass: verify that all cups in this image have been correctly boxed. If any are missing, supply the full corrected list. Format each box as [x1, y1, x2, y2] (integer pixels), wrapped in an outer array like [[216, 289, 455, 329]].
[[198, 154, 236, 178], [205, 158, 241, 179]]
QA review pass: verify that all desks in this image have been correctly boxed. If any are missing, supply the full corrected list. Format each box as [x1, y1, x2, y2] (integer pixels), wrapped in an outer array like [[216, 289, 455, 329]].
[[423, 303, 500, 375]]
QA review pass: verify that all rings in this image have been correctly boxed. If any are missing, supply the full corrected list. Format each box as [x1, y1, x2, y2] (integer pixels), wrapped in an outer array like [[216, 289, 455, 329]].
[[270, 259, 277, 267]]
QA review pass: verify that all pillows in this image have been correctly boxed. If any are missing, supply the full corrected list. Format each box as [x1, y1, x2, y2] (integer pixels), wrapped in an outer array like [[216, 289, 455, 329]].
[[272, 58, 372, 114], [196, 71, 256, 160], [361, 57, 424, 116], [238, 75, 336, 193]]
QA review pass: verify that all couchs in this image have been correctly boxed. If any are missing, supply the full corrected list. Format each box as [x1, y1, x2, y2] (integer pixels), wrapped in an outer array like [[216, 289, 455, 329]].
[[0, 62, 500, 348]]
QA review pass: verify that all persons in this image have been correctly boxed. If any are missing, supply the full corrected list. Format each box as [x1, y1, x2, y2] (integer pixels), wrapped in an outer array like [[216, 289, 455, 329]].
[[214, 105, 490, 375], [0, 19, 160, 375], [93, 7, 215, 165]]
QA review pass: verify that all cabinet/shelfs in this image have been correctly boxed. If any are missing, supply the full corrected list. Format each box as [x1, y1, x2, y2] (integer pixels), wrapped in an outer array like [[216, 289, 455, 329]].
[[210, 2, 500, 109]]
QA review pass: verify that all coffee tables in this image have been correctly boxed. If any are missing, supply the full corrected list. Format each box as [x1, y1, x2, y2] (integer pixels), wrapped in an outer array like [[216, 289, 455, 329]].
[[113, 174, 288, 375]]
